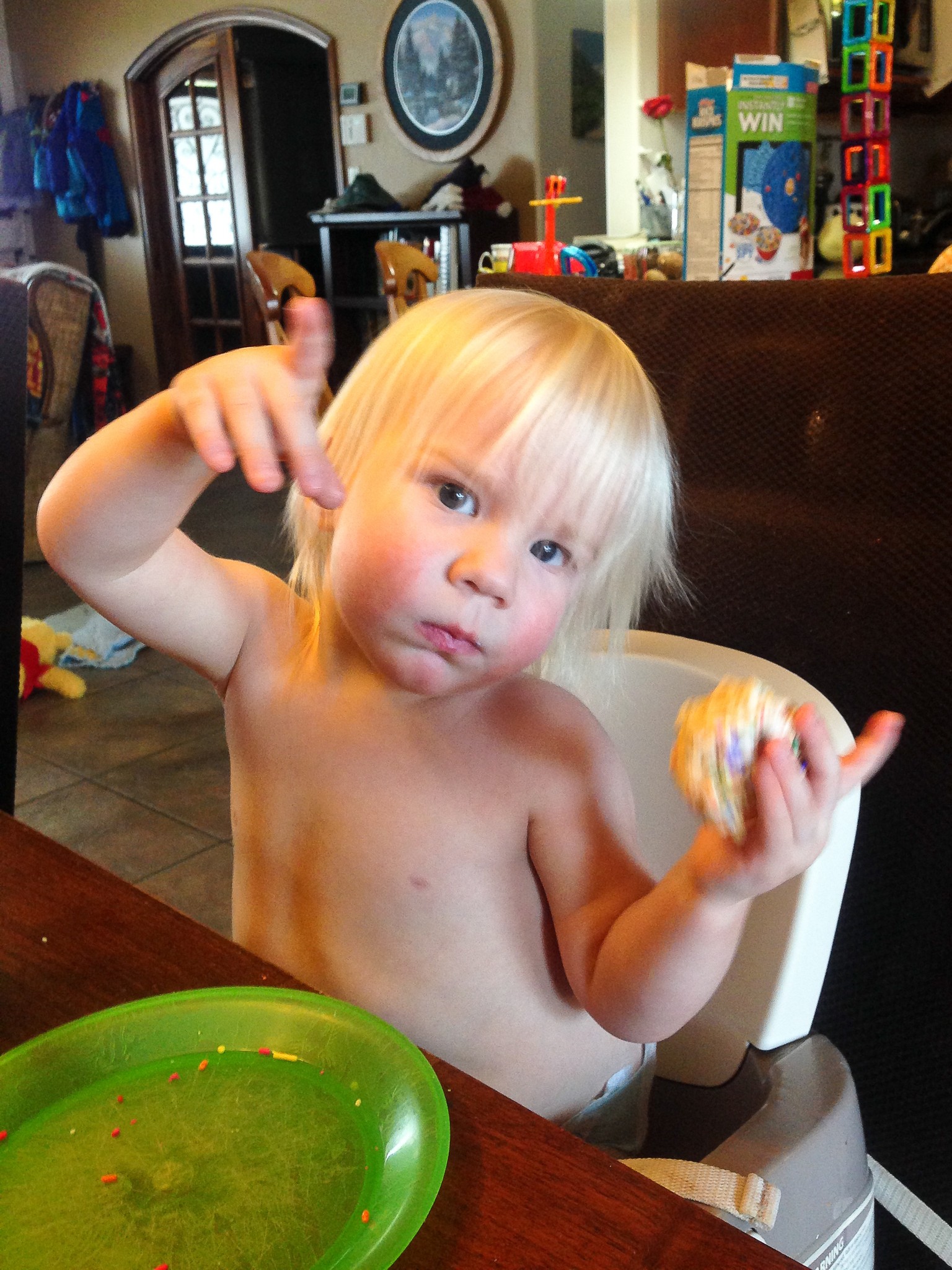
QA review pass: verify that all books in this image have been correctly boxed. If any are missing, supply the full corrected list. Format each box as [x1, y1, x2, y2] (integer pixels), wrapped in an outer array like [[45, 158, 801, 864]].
[[375, 223, 458, 294]]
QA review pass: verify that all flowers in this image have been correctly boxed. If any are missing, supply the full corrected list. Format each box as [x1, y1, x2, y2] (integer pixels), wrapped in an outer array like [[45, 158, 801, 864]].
[[641, 92, 680, 191]]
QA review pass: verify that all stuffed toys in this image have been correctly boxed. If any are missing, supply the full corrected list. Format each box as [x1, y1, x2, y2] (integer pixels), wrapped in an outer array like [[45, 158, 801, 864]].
[[19, 616, 85, 699]]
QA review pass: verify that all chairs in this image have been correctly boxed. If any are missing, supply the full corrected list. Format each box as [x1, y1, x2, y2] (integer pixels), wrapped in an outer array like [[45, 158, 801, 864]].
[[524, 626, 876, 1270], [242, 241, 442, 420]]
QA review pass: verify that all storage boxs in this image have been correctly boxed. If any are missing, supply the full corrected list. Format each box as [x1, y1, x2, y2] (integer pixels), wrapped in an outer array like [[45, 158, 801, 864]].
[[681, 51, 820, 281]]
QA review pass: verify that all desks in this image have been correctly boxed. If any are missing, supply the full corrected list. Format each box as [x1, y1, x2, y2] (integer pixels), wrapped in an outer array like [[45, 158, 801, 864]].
[[1, 811, 811, 1270]]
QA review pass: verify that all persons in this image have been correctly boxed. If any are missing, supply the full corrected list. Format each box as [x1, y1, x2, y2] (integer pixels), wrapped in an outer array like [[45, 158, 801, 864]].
[[36, 286, 904, 1150]]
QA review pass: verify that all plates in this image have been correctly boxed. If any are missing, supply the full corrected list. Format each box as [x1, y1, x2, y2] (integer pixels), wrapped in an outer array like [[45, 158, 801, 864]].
[[0, 985, 451, 1270]]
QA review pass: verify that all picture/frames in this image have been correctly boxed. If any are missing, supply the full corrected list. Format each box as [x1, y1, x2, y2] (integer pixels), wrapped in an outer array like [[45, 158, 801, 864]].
[[376, 0, 505, 163]]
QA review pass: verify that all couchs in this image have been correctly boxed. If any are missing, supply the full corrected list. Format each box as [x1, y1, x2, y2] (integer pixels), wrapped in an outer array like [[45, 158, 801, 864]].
[[474, 268, 952, 1113], [1, 263, 101, 564]]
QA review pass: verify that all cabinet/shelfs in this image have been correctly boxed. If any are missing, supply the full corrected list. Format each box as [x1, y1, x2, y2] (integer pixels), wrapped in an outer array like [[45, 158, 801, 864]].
[[657, 0, 782, 111], [307, 209, 519, 393]]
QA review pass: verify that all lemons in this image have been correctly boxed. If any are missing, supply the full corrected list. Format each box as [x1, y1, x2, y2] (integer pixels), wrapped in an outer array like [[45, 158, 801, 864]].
[[816, 212, 867, 280], [642, 250, 684, 281]]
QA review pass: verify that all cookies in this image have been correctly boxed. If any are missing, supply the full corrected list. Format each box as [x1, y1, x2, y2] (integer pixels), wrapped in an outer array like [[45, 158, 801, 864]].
[[670, 675, 812, 840]]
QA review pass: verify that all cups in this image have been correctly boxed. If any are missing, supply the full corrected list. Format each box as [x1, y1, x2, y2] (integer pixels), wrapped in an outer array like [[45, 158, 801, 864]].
[[640, 204, 672, 241], [478, 243, 513, 274]]
